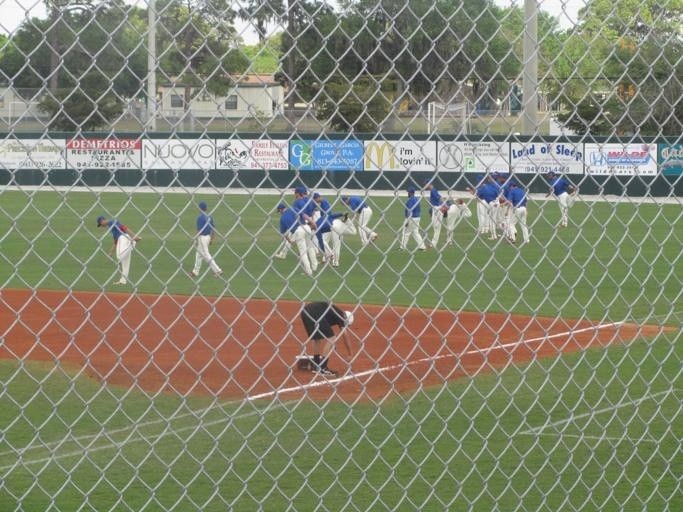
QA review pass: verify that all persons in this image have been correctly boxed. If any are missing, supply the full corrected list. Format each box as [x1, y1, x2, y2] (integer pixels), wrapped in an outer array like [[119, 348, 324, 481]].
[[547, 172, 578, 227], [276, 186, 358, 277], [425, 183, 473, 248], [299, 301, 354, 375], [340, 196, 377, 249], [188, 201, 223, 278], [476, 174, 531, 243], [97, 215, 139, 285], [397, 185, 428, 252]]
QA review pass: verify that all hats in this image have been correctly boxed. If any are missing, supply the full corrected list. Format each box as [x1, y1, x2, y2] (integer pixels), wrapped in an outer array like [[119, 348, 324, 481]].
[[277, 203, 285, 209], [423, 177, 432, 184], [344, 310, 354, 326], [97, 216, 105, 227], [199, 202, 207, 209], [547, 171, 555, 175], [313, 193, 319, 199], [292, 186, 307, 193], [406, 186, 415, 192], [428, 207, 432, 217], [476, 171, 516, 187]]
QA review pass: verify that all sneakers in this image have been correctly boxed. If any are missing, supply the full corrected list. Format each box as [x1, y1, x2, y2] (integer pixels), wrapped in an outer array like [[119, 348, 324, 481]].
[[301, 251, 340, 275], [341, 212, 349, 222], [213, 271, 223, 278], [112, 281, 126, 286], [481, 229, 530, 243], [396, 239, 454, 252], [560, 224, 567, 227], [187, 271, 194, 276], [312, 366, 336, 376], [370, 232, 378, 244], [275, 251, 287, 259]]
